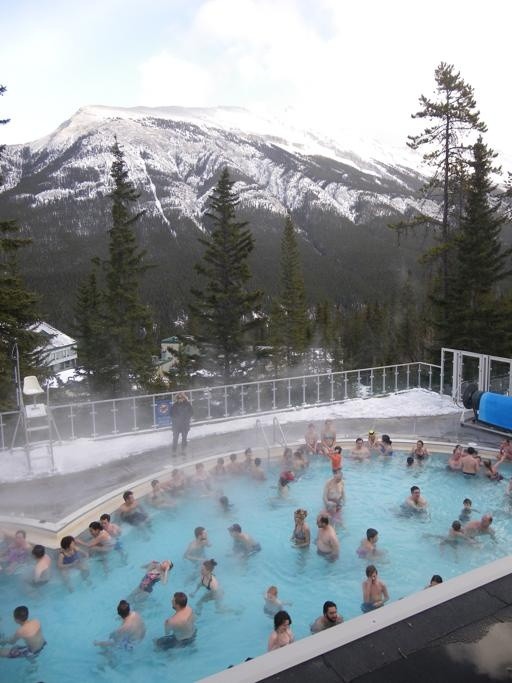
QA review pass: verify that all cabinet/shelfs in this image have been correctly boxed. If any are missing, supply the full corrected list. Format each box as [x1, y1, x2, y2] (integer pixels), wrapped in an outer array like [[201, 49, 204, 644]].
[[22, 404, 54, 469]]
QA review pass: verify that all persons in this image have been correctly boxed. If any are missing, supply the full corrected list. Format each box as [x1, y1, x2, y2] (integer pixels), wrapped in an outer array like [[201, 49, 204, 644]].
[[8, 606, 47, 653], [362, 564, 391, 607], [183, 522, 284, 617], [171, 393, 194, 458], [164, 447, 268, 512], [116, 598, 146, 641], [291, 470, 379, 565], [268, 609, 295, 650], [273, 419, 395, 491], [2, 491, 173, 600], [313, 600, 343, 632], [163, 589, 198, 641], [401, 432, 512, 544], [426, 574, 444, 589]]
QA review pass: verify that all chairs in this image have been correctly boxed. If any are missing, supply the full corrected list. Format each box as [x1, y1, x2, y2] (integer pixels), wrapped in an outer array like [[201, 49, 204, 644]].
[[22, 375, 45, 410]]
